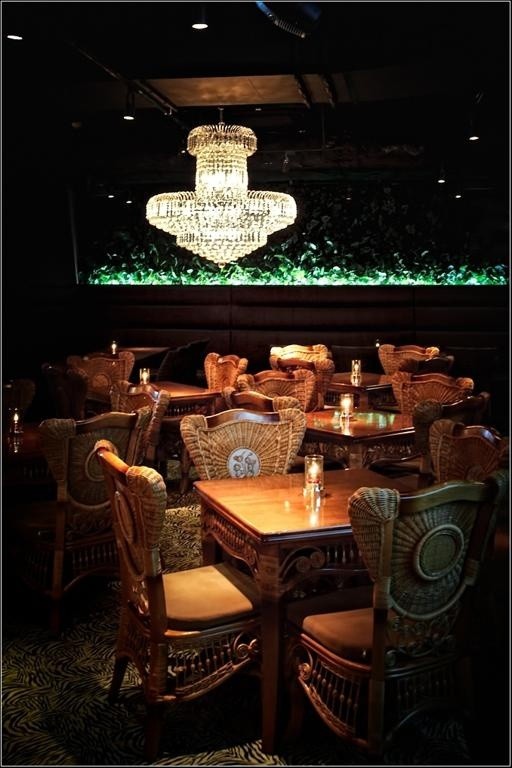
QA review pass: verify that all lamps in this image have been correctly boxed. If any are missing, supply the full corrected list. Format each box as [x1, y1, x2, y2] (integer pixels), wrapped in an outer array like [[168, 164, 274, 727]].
[[145, 105, 299, 271]]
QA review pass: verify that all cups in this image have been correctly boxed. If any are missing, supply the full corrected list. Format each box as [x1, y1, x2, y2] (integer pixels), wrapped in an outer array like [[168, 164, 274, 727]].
[[140, 367, 150, 385], [110, 340, 117, 355], [351, 360, 362, 386], [340, 393, 356, 418], [304, 455, 326, 496], [6, 404, 26, 434]]
